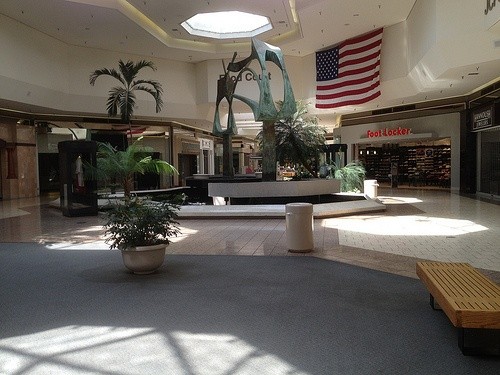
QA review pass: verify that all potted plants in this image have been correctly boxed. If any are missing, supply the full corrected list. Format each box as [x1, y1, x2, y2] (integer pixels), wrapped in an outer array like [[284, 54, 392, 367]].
[[98, 191, 183, 275]]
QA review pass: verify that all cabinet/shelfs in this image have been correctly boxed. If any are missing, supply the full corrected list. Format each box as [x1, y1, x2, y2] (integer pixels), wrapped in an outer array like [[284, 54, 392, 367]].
[[358, 144, 451, 187]]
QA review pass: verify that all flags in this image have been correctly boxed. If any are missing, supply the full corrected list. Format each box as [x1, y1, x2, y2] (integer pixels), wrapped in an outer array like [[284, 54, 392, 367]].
[[314, 27, 384, 108]]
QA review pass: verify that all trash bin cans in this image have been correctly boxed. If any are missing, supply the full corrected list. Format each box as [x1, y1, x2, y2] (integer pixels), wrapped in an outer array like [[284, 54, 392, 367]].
[[285, 202, 315, 252], [364, 178, 380, 201]]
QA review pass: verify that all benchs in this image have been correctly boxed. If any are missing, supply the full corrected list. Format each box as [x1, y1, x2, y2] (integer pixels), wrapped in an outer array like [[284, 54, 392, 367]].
[[415, 261, 500, 356]]
